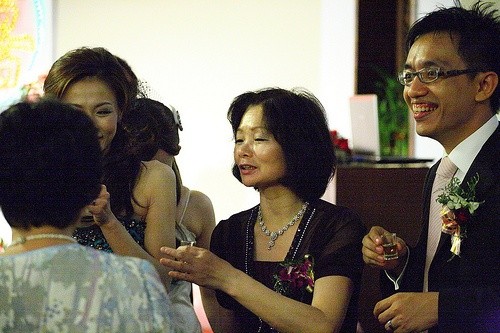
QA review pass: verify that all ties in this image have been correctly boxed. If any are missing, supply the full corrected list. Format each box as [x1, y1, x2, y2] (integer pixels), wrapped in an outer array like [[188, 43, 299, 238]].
[[423, 156, 458, 292]]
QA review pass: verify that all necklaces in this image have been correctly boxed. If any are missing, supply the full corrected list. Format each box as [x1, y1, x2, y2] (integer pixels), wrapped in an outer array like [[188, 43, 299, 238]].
[[258, 201, 308, 249], [6, 233, 78, 249]]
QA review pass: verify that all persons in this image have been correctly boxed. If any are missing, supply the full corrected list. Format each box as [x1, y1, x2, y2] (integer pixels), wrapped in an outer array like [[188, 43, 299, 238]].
[[361, 1, 500, 333], [160, 87, 367, 333], [0, 48, 217, 333]]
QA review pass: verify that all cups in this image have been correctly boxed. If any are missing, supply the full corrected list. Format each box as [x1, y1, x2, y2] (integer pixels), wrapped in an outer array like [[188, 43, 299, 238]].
[[380, 233, 398, 261], [181, 240, 196, 247]]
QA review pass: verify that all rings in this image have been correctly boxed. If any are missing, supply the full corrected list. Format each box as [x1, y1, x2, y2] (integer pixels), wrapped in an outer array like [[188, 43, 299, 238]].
[[93, 199, 96, 206], [387, 320, 395, 332]]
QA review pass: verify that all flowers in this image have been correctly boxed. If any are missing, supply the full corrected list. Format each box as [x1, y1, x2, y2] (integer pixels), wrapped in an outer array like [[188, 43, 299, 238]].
[[273, 255, 315, 296], [432, 172, 486, 264]]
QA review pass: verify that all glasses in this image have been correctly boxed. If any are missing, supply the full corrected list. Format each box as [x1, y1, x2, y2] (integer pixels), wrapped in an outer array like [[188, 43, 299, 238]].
[[396, 67, 491, 85]]
